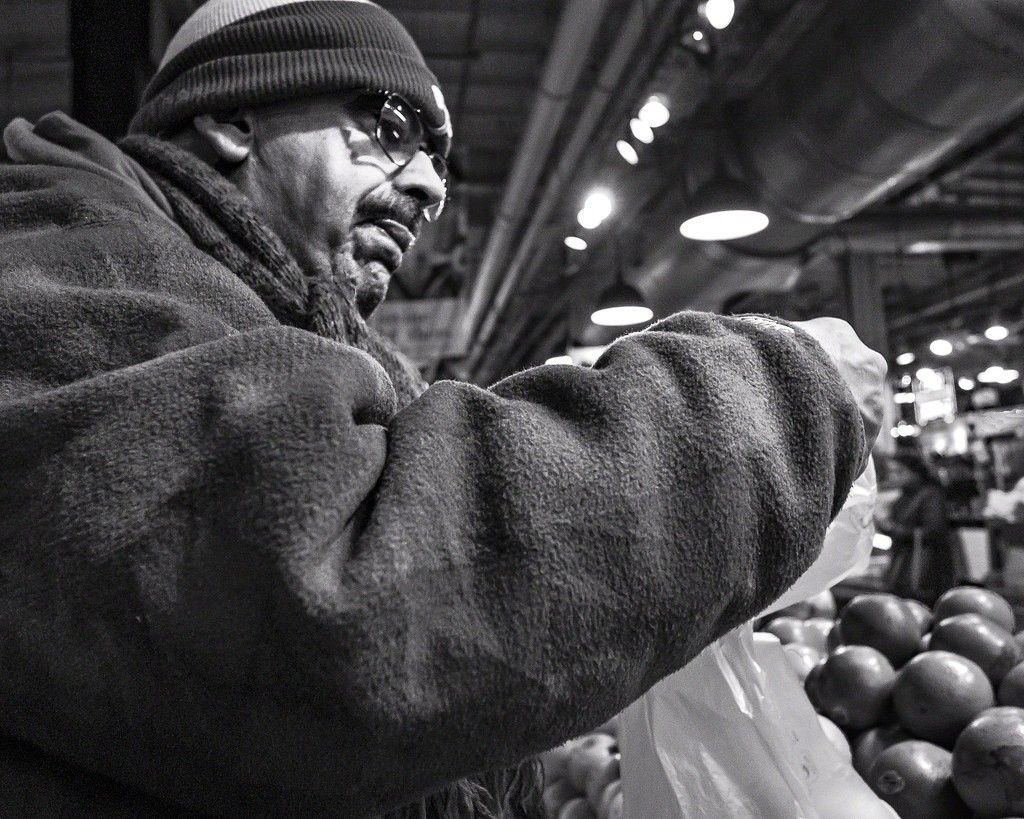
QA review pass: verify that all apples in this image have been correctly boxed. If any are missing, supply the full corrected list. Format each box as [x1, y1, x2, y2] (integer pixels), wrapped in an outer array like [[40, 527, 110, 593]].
[[534, 733, 624, 819]]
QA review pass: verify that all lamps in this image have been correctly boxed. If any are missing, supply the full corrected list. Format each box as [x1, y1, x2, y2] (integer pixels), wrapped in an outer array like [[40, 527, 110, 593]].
[[678, 150, 769, 243], [984, 291, 1009, 341], [590, 240, 654, 327]]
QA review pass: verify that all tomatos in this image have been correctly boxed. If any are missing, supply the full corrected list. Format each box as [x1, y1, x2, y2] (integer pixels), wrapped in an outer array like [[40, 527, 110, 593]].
[[758, 586, 1024, 819]]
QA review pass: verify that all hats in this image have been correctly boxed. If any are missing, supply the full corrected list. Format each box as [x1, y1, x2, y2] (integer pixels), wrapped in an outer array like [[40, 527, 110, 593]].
[[129, 0, 454, 160]]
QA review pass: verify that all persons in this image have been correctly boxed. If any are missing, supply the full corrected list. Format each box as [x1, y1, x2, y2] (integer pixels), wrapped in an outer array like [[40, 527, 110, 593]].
[[2, 3, 890, 819], [873, 450, 959, 605]]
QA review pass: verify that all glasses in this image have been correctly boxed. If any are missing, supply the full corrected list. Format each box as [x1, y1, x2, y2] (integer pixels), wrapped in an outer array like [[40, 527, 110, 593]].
[[352, 86, 449, 224]]
